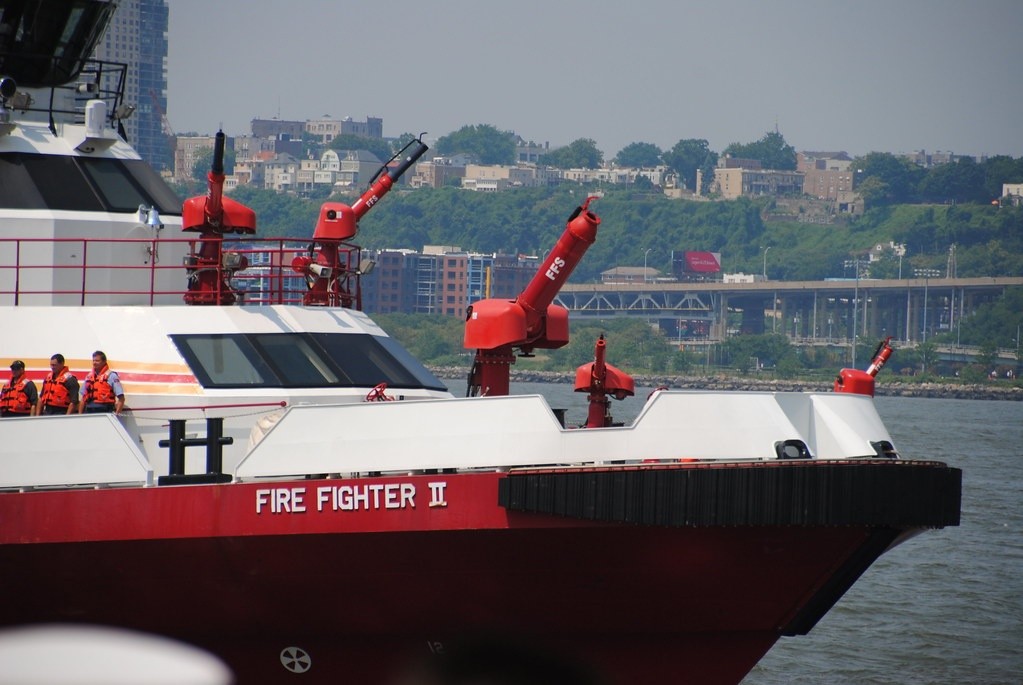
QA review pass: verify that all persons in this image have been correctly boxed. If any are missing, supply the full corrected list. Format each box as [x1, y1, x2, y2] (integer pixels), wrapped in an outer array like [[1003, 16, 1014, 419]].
[[0, 360, 38, 416], [78, 351, 125, 415], [37, 354, 80, 415]]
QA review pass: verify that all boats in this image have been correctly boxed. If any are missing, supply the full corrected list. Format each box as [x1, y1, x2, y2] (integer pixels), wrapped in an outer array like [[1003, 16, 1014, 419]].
[[1, 0, 964, 683]]
